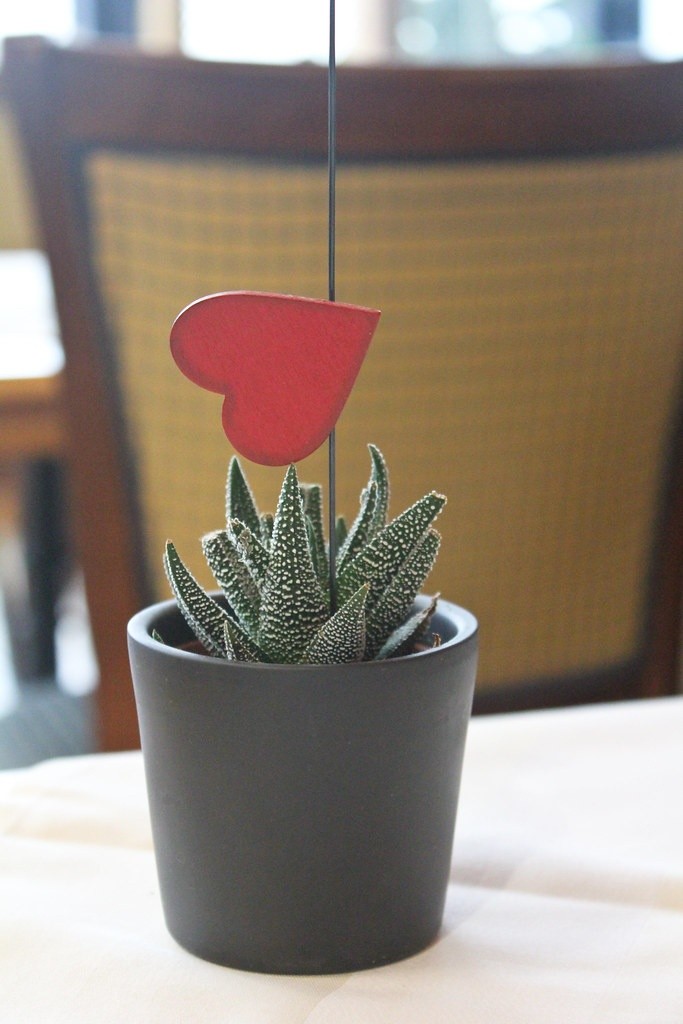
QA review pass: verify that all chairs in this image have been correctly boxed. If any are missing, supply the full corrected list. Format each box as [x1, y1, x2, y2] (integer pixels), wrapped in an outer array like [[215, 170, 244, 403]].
[[7, 36, 683, 753]]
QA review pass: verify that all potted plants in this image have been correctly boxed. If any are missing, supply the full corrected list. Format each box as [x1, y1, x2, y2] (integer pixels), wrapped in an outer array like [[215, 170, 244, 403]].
[[124, 443, 482, 977]]
